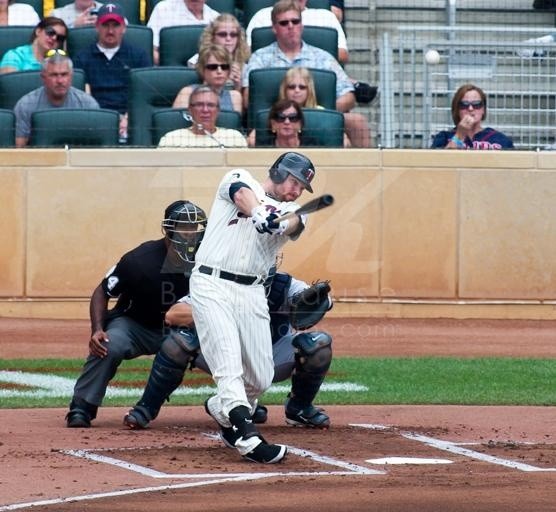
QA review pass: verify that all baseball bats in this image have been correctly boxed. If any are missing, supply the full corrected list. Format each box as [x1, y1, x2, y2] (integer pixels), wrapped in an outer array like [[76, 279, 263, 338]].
[[272, 196, 334, 223]]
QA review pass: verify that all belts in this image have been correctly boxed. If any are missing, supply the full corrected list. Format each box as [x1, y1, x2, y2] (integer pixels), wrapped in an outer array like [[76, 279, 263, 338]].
[[198, 265, 276, 288]]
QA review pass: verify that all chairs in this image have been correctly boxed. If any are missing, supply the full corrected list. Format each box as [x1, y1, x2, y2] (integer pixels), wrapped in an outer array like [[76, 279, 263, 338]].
[[0, 1, 347, 150]]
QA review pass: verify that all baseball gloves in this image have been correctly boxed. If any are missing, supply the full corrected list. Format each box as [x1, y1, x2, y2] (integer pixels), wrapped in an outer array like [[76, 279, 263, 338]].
[[289, 281, 330, 329]]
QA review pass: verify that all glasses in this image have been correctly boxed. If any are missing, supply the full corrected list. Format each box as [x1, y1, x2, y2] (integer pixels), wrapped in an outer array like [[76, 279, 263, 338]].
[[272, 112, 300, 123], [278, 19, 301, 26], [287, 83, 307, 90], [44, 26, 65, 43], [213, 30, 237, 39], [44, 48, 67, 57], [457, 101, 483, 110], [203, 62, 230, 72]]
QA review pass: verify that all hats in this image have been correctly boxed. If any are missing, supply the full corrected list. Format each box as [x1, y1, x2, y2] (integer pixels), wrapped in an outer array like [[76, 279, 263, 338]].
[[96, 2, 125, 27]]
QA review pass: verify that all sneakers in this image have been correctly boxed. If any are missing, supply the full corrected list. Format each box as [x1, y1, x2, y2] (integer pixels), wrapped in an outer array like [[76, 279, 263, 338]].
[[124, 405, 154, 430], [354, 81, 381, 108], [204, 398, 331, 464], [66, 406, 91, 428]]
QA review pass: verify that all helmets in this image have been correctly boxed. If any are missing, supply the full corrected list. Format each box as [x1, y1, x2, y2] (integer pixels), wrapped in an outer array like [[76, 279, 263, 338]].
[[269, 151, 316, 194], [161, 199, 206, 264]]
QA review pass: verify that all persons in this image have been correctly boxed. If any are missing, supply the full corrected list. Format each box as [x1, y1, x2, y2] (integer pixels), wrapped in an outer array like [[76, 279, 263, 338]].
[[64, 200, 211, 430], [189, 151, 316, 464], [430, 84, 512, 151], [122, 255, 333, 427]]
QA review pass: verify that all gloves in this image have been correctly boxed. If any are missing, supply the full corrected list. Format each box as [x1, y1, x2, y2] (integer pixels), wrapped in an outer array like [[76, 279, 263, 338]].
[[251, 204, 289, 236]]
